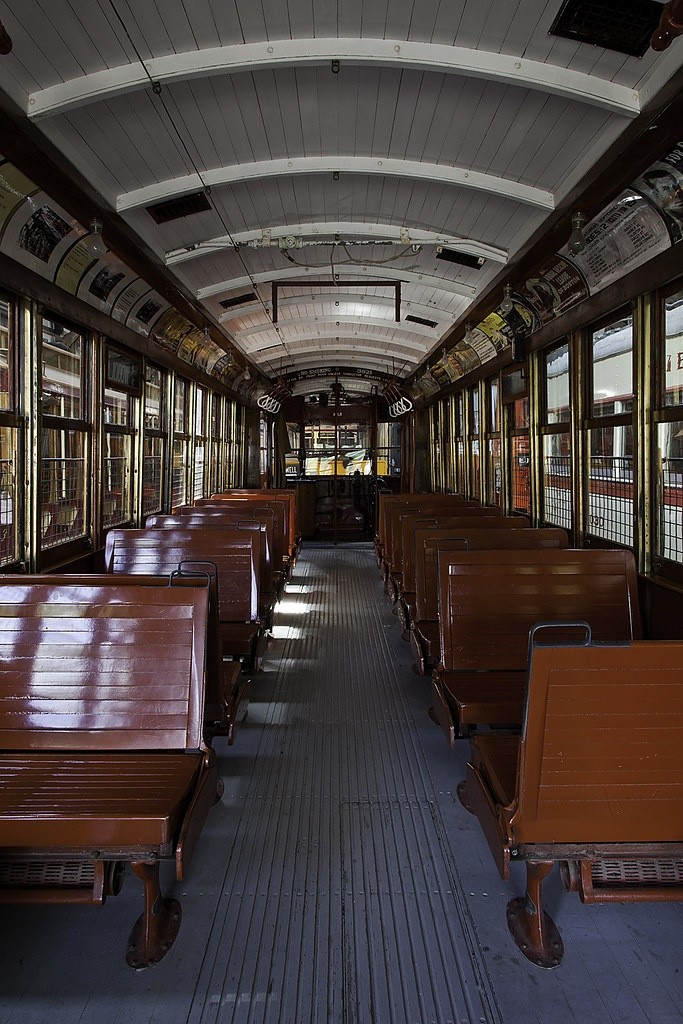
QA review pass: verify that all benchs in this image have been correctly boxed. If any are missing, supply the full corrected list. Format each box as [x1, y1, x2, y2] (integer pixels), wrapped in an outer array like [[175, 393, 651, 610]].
[[0, 488, 300, 956], [376, 493, 683, 967]]
